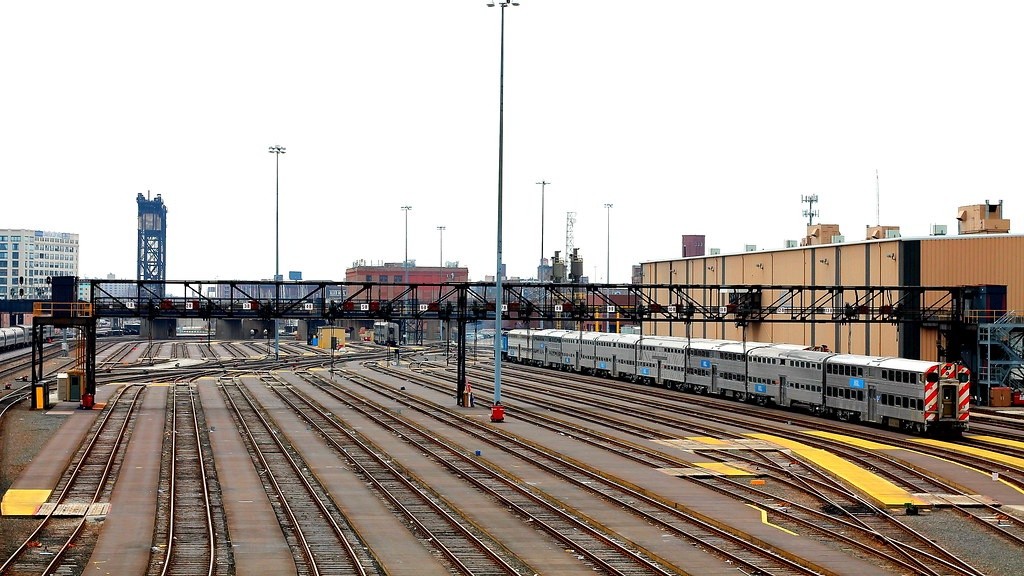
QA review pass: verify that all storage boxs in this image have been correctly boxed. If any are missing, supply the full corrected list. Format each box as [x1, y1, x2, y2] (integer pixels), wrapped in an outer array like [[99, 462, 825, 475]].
[[991, 387, 1011, 406]]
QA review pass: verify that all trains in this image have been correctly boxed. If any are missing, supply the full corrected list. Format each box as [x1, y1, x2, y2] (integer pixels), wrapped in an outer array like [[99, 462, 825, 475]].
[[0, 323, 55, 354], [500, 327, 972, 440]]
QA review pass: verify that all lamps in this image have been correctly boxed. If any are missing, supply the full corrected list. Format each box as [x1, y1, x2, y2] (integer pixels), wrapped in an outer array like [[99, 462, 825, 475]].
[[756, 263, 764, 270], [708, 265, 714, 272], [670, 269, 676, 274], [639, 272, 645, 276], [887, 252, 896, 261], [819, 259, 828, 264]]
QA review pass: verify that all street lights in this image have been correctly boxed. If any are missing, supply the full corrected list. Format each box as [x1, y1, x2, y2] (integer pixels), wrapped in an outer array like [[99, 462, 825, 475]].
[[401, 205, 413, 344], [485, 0, 522, 405], [268, 144, 286, 361], [604, 203, 614, 333], [536, 181, 552, 330], [436, 226, 446, 340]]
